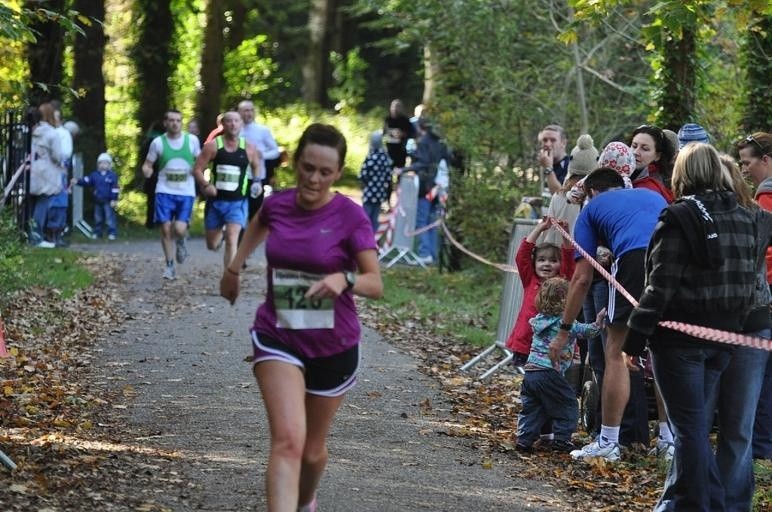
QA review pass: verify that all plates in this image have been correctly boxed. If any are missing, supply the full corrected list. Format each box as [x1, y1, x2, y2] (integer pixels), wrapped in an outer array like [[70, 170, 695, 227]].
[[513, 218, 543, 225]]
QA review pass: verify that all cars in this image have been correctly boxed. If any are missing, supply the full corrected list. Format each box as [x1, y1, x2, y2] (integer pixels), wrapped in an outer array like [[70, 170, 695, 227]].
[[369, 116, 468, 178]]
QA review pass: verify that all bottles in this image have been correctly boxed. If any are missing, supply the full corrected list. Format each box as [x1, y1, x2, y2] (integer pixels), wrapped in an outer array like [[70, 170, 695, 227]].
[[541, 188, 551, 218], [250, 178, 259, 199]]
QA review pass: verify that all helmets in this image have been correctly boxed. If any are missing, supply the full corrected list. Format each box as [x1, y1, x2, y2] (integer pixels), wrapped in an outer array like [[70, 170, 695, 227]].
[[566, 135, 601, 176]]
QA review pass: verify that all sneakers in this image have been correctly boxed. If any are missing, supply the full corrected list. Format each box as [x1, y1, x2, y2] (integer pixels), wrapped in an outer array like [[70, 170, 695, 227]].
[[514, 444, 534, 454], [646, 435, 675, 463], [90, 232, 117, 241], [30, 238, 71, 251], [552, 439, 581, 453], [175, 237, 188, 263], [568, 434, 622, 462], [408, 254, 434, 266], [161, 265, 177, 280]]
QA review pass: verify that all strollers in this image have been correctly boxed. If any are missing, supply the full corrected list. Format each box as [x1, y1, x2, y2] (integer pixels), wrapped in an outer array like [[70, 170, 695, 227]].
[[577, 308, 661, 445]]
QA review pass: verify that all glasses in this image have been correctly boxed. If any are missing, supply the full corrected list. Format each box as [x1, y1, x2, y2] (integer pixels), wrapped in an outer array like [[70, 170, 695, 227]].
[[746, 134, 765, 150], [636, 124, 664, 145]]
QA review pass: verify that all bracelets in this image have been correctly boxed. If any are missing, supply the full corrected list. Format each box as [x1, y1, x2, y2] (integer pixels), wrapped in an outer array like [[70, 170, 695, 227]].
[[228, 268, 240, 276]]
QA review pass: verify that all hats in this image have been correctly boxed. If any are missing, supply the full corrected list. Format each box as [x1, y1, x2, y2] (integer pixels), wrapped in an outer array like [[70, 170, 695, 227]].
[[408, 103, 433, 127], [96, 152, 114, 165], [369, 131, 383, 148], [661, 129, 680, 157], [677, 122, 710, 151]]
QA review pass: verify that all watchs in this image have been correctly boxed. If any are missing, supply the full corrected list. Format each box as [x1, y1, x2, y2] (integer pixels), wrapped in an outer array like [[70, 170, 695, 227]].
[[344, 271, 355, 289]]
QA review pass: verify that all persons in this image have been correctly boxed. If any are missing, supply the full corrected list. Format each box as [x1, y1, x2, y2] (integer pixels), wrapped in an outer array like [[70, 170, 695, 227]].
[[204, 113, 225, 146], [142, 107, 201, 281], [358, 98, 472, 274], [6, 98, 120, 250], [186, 119, 205, 149], [221, 124, 384, 511], [237, 100, 280, 269], [192, 111, 263, 273]]
